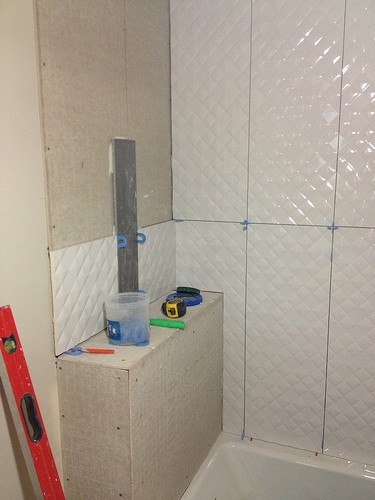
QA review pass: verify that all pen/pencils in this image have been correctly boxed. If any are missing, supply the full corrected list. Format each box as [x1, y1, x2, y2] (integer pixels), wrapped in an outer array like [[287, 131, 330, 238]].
[[77, 348, 115, 354]]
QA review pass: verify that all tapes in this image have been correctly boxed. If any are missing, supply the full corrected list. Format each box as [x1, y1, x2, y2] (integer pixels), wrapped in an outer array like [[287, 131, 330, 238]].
[[165, 292, 202, 306]]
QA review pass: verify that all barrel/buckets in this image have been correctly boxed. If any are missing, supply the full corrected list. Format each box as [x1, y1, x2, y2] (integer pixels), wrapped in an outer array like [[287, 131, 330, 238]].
[[105, 291, 151, 346]]
